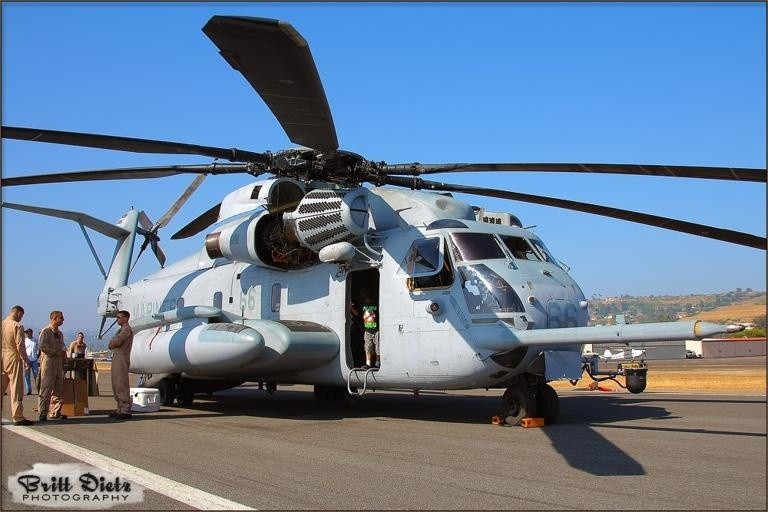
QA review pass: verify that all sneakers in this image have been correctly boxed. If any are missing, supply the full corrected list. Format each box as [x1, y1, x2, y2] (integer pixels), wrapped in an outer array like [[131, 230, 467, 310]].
[[361, 365, 370, 369], [13, 420, 35, 426], [39, 414, 67, 422], [109, 413, 133, 420]]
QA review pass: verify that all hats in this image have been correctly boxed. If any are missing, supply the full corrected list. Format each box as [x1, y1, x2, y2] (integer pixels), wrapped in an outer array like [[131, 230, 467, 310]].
[[24, 328, 33, 333]]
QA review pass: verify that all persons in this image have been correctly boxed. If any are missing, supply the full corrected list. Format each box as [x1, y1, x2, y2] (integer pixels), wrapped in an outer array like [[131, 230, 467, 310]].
[[106, 311, 134, 419], [68, 331, 87, 358], [1, 304, 34, 424], [22, 328, 41, 395], [350, 295, 379, 368], [36, 310, 68, 421]]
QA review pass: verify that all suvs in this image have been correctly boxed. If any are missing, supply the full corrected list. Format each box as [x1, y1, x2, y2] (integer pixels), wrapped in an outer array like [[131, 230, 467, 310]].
[[582, 349, 600, 363]]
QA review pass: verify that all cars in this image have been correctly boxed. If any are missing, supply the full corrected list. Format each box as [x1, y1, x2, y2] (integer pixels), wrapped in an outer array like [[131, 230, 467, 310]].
[[686, 349, 696, 359]]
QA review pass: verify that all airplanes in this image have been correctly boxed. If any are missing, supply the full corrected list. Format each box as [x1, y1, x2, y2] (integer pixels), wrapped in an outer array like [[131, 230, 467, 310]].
[[599, 343, 649, 364]]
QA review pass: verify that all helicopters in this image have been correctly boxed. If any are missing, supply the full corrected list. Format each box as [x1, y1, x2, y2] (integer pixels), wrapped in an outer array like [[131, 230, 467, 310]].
[[3, 17, 767, 429]]
